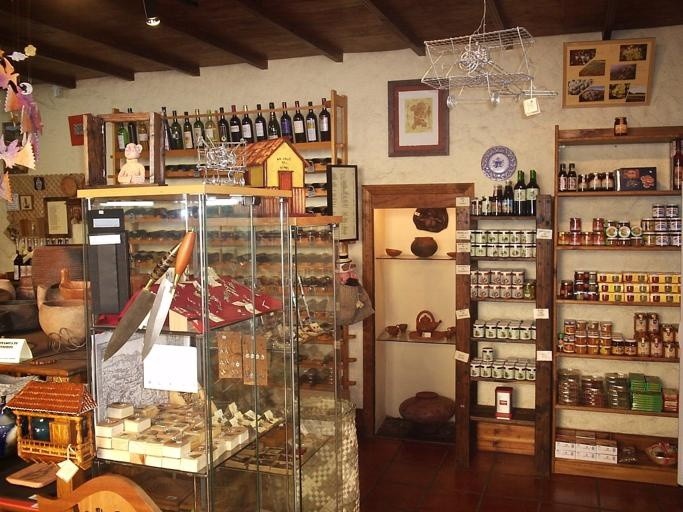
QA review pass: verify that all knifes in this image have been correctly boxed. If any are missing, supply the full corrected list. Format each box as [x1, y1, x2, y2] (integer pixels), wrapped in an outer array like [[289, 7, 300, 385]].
[[102, 229, 197, 362]]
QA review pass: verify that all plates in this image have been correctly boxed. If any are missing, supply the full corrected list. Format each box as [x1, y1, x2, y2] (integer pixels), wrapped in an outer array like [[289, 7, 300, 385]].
[[447, 252, 456, 259], [480, 147, 517, 182]]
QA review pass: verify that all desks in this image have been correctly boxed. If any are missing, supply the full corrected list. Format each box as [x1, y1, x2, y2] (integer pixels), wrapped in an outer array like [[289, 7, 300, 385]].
[[0, 332, 87, 443]]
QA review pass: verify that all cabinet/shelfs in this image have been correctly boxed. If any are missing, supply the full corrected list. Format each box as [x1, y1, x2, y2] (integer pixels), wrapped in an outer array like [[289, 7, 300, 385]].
[[552, 123, 683, 486], [362, 181, 475, 457], [454, 195, 551, 475], [82, 89, 357, 402]]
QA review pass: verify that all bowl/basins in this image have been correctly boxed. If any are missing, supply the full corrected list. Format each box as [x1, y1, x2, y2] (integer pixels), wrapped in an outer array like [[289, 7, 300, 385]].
[[37, 299, 90, 345], [386, 249, 401, 257], [645, 444, 677, 466]]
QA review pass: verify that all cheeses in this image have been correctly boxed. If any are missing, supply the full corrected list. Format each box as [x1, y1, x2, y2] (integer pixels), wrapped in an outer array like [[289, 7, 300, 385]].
[[95, 400, 249, 472]]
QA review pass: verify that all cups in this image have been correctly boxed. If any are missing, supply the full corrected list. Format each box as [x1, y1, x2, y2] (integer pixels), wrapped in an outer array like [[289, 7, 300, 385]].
[[472, 196, 492, 215], [383, 326, 399, 336], [396, 324, 407, 332]]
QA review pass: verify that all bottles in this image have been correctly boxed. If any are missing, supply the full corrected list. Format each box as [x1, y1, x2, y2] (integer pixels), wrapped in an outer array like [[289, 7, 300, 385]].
[[115, 96, 330, 154], [492, 169, 539, 216], [12, 237, 69, 281], [567, 163, 576, 192], [300, 360, 329, 387], [671, 140, 682, 191], [559, 163, 567, 192], [125, 206, 331, 308]]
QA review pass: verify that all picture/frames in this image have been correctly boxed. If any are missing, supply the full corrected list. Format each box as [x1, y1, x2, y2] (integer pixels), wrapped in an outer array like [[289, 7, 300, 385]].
[[43, 197, 71, 238], [18, 194, 33, 211], [386, 77, 450, 157], [562, 37, 656, 109]]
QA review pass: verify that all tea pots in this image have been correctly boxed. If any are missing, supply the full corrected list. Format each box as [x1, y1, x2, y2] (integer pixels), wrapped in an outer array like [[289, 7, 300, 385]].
[[415, 310, 441, 335]]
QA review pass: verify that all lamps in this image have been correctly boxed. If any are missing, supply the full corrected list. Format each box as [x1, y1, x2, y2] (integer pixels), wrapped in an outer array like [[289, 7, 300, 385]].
[[141, 1, 161, 28]]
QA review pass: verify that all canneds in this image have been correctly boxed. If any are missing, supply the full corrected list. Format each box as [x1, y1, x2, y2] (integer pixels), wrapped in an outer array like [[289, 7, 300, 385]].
[[470, 230, 536, 258], [471, 319, 537, 339], [557, 203, 682, 248], [472, 196, 491, 215], [471, 270, 536, 299], [574, 272, 598, 301], [564, 313, 680, 358], [470, 348, 536, 380], [561, 281, 573, 299], [613, 117, 629, 135], [578, 171, 615, 189]]
